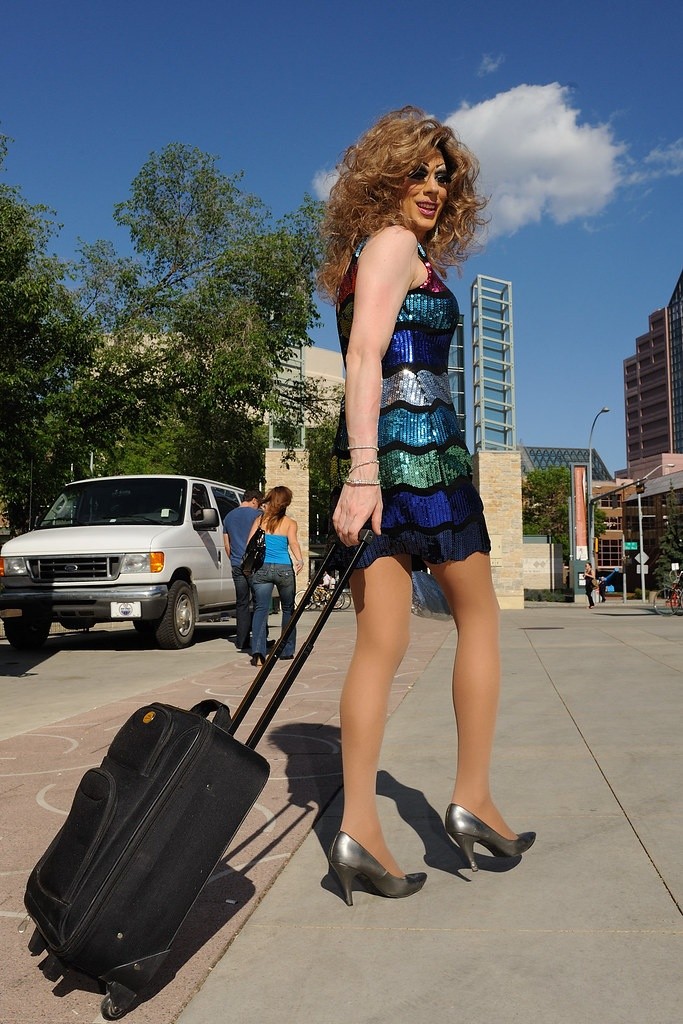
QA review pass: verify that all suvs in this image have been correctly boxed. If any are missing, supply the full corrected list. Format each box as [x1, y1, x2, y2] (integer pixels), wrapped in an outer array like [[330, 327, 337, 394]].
[[0, 474, 279, 649]]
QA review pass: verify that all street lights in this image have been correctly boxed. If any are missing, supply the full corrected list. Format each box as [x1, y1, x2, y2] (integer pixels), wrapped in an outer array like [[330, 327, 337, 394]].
[[588, 407, 613, 605], [636, 463, 676, 602]]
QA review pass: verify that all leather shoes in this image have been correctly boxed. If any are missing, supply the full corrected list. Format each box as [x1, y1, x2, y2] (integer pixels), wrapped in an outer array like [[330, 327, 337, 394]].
[[266, 639, 276, 648]]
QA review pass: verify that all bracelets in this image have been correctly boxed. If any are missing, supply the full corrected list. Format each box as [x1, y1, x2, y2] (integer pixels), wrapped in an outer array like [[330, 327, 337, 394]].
[[342, 446, 381, 486]]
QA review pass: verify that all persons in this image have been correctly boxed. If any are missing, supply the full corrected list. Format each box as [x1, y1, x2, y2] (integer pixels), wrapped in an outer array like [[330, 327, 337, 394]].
[[319, 107, 535, 908], [321, 571, 336, 593], [583, 564, 595, 609], [223, 486, 304, 666]]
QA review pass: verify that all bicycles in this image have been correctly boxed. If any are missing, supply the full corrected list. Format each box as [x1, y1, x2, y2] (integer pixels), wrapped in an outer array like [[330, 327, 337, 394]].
[[294, 580, 351, 610], [654, 567, 683, 617]]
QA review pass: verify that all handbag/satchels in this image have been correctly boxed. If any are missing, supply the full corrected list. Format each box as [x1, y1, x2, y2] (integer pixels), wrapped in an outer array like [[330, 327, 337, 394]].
[[240, 513, 266, 575], [590, 569, 598, 590]]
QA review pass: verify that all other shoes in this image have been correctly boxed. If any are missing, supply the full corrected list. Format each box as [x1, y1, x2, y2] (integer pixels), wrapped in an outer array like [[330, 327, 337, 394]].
[[589, 606, 594, 609]]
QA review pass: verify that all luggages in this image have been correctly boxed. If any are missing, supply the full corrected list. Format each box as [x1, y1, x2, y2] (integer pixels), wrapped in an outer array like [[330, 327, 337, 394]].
[[18, 529, 375, 1021]]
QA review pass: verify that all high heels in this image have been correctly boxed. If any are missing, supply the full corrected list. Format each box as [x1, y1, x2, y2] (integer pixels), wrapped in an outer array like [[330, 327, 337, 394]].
[[253, 654, 264, 666], [445, 803, 537, 872], [280, 655, 294, 660], [328, 830, 427, 906]]
